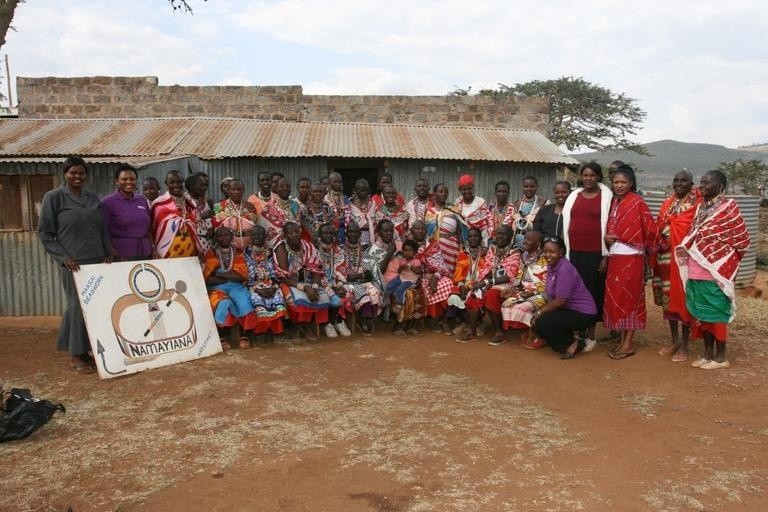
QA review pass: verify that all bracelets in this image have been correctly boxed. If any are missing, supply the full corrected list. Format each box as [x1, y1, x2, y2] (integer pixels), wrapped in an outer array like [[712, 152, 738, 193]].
[[151, 244, 155, 249]]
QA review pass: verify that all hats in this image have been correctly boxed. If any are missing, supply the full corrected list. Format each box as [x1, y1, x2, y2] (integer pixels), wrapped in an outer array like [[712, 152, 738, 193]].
[[457, 175, 474, 190], [220, 176, 234, 189]]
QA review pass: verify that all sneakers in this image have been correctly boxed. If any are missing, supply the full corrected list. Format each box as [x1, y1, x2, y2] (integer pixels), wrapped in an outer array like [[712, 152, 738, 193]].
[[68, 355, 97, 375], [691, 357, 712, 368], [700, 359, 730, 370]]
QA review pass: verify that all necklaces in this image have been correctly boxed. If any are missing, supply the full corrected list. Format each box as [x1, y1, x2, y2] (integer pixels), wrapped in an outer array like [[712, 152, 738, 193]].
[[663, 192, 690, 223], [699, 194, 722, 209]]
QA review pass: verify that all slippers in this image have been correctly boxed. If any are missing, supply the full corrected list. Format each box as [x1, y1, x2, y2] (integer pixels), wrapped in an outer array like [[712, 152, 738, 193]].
[[656, 345, 678, 358], [671, 352, 689, 362]]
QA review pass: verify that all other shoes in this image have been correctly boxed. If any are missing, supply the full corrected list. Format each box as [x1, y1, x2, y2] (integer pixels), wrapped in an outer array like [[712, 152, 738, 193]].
[[358, 318, 506, 346], [212, 322, 353, 351], [519, 334, 634, 360]]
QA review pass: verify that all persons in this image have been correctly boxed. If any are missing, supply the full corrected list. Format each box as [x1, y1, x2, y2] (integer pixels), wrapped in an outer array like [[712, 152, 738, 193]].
[[99, 165, 159, 262], [673, 169, 751, 371], [649, 167, 704, 362], [144, 159, 664, 360], [35, 155, 116, 374]]
